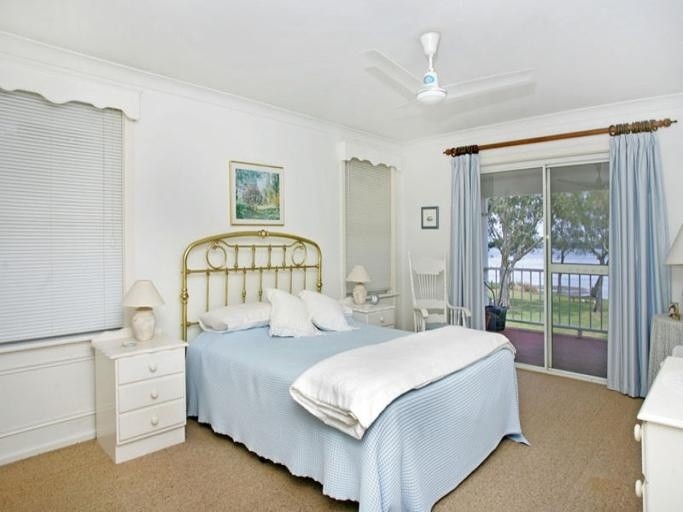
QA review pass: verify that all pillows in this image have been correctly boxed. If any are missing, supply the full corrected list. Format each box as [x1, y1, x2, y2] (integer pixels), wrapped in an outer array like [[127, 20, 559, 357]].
[[197, 301, 271, 334], [298, 288, 354, 333], [264, 287, 324, 337]]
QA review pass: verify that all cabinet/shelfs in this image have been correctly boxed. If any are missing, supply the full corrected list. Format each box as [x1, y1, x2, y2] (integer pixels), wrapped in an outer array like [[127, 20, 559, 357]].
[[631, 357, 681, 512]]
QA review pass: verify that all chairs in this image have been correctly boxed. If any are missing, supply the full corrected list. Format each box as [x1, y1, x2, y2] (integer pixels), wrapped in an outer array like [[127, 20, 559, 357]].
[[405, 249, 471, 332]]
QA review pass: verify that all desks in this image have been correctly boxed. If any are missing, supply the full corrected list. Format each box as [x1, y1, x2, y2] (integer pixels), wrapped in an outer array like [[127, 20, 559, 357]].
[[646, 312, 682, 392]]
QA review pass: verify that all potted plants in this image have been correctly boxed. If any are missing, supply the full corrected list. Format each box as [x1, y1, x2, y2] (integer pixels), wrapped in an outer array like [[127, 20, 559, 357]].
[[483, 261, 509, 332]]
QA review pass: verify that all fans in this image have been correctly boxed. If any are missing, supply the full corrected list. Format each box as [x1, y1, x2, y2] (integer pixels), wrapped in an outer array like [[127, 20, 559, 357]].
[[360, 31, 539, 118]]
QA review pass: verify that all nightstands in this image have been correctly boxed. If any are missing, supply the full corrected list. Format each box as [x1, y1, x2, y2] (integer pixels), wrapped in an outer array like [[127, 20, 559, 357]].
[[91, 331, 188, 466], [348, 304, 395, 328]]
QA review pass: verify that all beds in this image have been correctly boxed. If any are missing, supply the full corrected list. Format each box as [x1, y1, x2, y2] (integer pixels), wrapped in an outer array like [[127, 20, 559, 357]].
[[179, 229, 531, 512]]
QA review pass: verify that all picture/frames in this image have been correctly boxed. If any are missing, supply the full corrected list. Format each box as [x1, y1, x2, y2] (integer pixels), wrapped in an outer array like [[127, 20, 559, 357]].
[[420, 206, 439, 229], [228, 160, 284, 227]]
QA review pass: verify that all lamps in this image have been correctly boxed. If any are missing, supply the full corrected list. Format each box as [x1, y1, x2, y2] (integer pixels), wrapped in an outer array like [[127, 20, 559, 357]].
[[344, 264, 370, 305], [118, 279, 165, 341], [663, 224, 682, 268]]
